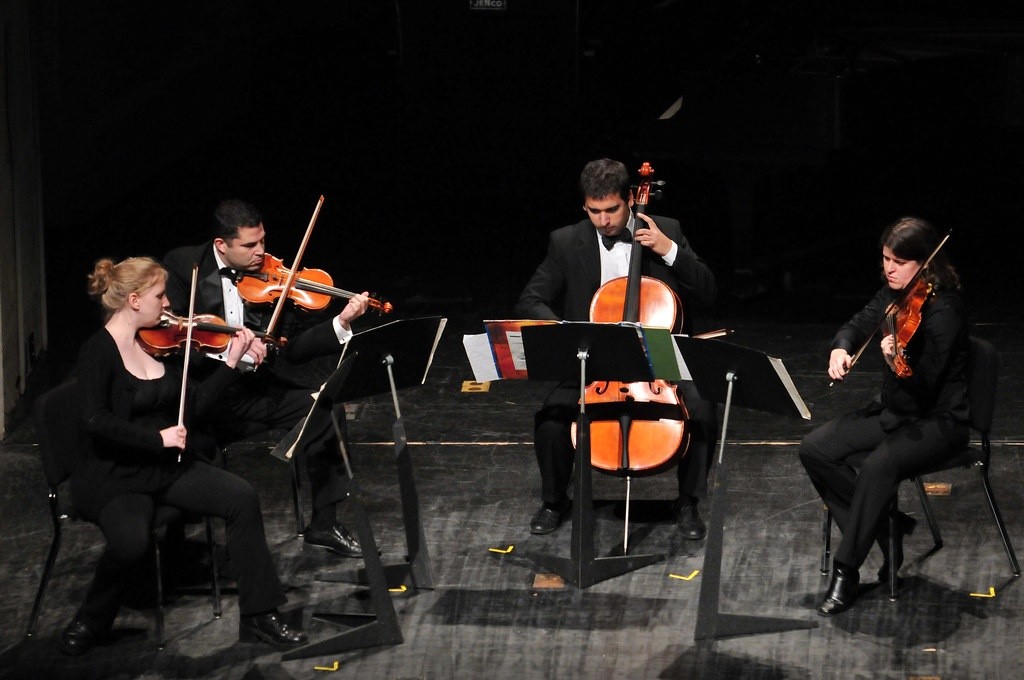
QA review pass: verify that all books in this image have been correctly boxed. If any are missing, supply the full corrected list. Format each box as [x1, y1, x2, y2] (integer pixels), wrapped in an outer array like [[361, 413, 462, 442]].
[[484, 319, 683, 381]]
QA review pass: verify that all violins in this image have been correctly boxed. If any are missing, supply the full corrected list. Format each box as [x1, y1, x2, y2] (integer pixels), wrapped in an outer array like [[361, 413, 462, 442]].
[[135, 309, 289, 358], [880, 276, 933, 381], [236, 252, 394, 314]]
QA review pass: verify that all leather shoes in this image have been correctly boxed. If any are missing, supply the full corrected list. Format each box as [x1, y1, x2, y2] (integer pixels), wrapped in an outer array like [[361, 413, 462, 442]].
[[304, 525, 382, 557], [530, 502, 565, 534], [673, 505, 706, 539]]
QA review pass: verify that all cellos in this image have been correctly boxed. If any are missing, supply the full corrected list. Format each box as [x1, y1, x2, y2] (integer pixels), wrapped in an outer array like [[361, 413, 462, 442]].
[[568, 160, 692, 479]]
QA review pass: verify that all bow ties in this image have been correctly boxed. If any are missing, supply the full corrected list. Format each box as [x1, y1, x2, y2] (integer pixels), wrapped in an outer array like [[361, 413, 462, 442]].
[[218, 267, 239, 285], [602, 227, 634, 251]]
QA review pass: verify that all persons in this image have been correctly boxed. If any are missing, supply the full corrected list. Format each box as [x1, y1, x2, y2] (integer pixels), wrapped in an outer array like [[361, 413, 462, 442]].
[[161, 202, 382, 557], [798, 219, 972, 616], [516, 159, 716, 541], [61, 257, 308, 657]]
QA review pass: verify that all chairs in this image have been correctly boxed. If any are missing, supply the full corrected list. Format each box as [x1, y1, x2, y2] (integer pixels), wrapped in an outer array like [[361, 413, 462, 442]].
[[819, 335, 1021, 602], [24, 381, 222, 648], [221, 429, 303, 533]]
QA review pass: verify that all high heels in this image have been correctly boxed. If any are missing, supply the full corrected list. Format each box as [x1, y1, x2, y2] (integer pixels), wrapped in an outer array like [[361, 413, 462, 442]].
[[817, 567, 861, 615], [238, 611, 308, 649], [877, 510, 917, 580], [61, 614, 94, 655]]
